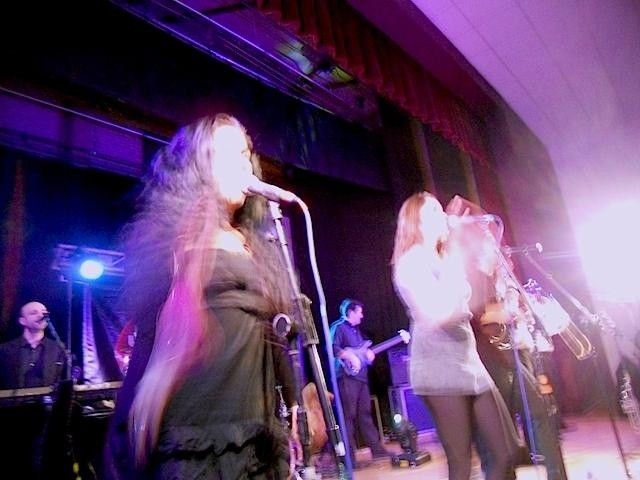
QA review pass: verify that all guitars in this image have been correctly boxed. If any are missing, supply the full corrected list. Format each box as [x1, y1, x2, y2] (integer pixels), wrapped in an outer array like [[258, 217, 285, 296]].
[[340, 330, 411, 375]]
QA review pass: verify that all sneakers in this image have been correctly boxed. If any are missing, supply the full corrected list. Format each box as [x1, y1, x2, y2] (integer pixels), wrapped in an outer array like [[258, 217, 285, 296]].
[[351, 450, 397, 470]]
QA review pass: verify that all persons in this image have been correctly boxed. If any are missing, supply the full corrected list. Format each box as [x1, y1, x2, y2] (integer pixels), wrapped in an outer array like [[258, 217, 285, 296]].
[[101, 108, 299, 477], [333, 299, 398, 469], [114, 316, 140, 382], [388, 190, 514, 480], [0, 302, 75, 479], [461, 219, 588, 465]]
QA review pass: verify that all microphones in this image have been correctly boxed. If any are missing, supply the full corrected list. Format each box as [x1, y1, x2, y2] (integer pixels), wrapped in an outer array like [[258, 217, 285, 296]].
[[497, 242, 543, 259], [447, 213, 492, 227], [241, 173, 299, 205], [38, 312, 50, 324]]
